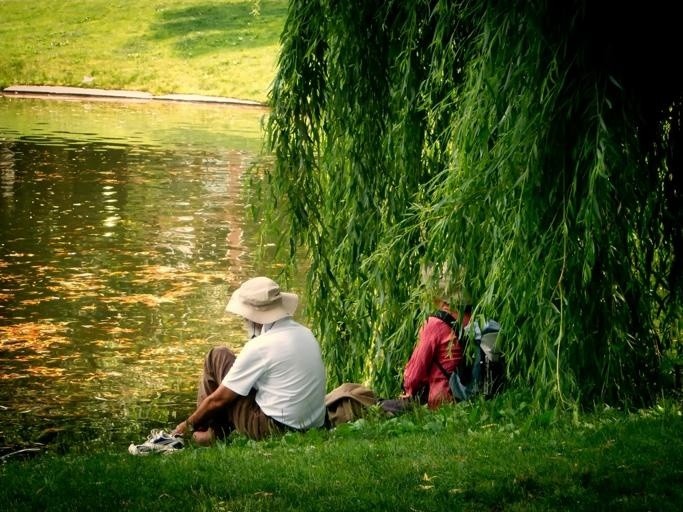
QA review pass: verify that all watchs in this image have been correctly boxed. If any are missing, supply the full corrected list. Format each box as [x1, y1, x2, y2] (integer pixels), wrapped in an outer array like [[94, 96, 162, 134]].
[[184, 420, 193, 432]]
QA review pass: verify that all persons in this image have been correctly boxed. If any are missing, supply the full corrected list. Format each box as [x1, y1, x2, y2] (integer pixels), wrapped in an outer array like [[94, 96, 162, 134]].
[[172, 276, 329, 443], [374, 259, 475, 420]]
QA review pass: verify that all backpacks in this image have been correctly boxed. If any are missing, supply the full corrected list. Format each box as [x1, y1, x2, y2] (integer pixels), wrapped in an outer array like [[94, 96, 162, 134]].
[[429, 310, 504, 403]]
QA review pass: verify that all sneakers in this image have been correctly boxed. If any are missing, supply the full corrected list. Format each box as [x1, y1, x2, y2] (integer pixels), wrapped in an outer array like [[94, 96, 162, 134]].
[[128, 429, 185, 456]]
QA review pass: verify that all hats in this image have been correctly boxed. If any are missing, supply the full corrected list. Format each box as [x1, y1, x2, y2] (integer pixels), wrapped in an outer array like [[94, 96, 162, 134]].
[[422, 262, 475, 306], [227, 277, 299, 323]]
